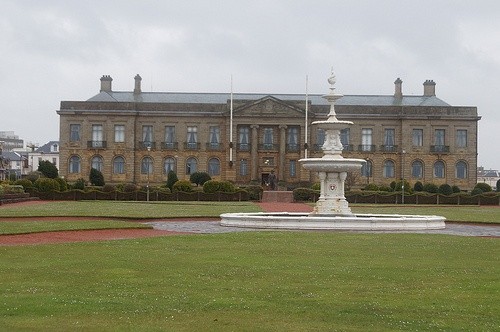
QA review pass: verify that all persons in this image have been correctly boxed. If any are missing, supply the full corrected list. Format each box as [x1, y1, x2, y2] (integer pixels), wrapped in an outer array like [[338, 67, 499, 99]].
[[268, 170, 276, 191]]
